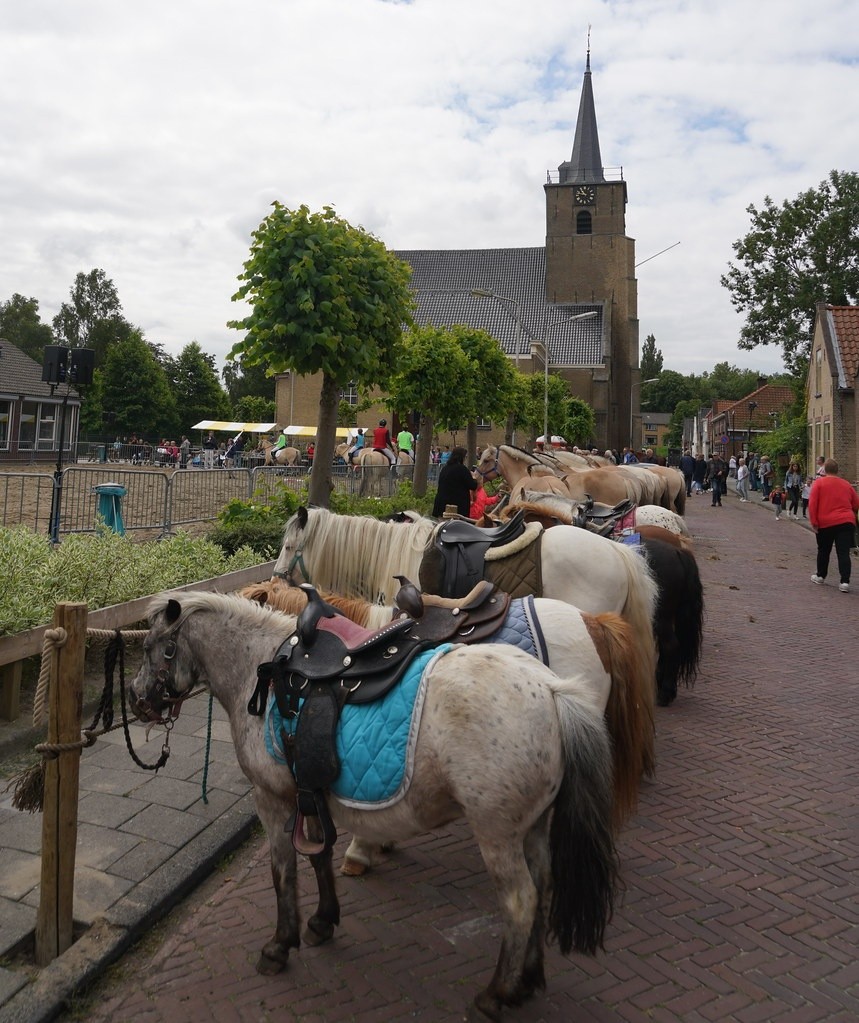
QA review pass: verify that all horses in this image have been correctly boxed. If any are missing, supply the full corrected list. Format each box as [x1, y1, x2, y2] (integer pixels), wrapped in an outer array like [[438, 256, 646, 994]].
[[124, 440, 706, 1023]]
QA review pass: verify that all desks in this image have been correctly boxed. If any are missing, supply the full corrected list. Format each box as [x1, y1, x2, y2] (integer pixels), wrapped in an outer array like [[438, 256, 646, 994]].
[[88, 446, 119, 463]]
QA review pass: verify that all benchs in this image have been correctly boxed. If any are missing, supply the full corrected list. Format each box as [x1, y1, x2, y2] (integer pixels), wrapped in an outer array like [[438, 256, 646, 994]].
[[86, 452, 119, 456]]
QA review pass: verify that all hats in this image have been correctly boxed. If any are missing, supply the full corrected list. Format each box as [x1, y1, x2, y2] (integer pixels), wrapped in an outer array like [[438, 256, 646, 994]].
[[712, 452, 719, 455]]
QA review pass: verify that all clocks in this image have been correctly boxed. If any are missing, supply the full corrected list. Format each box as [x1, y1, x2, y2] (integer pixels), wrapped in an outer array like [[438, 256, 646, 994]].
[[573, 185, 598, 206]]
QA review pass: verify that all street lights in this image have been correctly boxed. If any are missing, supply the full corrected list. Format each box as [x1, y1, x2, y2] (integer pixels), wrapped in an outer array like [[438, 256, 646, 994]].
[[543, 310, 599, 452], [629, 378, 660, 455]]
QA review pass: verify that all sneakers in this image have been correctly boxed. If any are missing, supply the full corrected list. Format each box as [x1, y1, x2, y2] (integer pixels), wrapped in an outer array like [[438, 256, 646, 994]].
[[810, 574, 824, 584], [839, 582, 849, 592]]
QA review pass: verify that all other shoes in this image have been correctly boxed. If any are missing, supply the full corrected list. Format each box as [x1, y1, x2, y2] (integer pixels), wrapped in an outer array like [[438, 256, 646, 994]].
[[803, 515, 807, 519], [775, 516, 779, 520], [762, 498, 769, 501], [740, 498, 749, 502], [687, 494, 691, 497], [794, 515, 800, 520], [751, 488, 757, 491], [787, 510, 791, 517], [711, 504, 716, 506], [719, 502, 722, 506]]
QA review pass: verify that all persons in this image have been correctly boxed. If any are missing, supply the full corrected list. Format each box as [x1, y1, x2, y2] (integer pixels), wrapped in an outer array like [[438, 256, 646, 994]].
[[678, 450, 729, 507], [158, 435, 194, 468], [591, 447, 659, 466], [348, 428, 364, 465], [808, 458, 859, 593], [307, 442, 315, 466], [431, 447, 478, 518], [202, 430, 218, 450], [396, 423, 415, 459], [271, 430, 286, 460], [108, 431, 156, 467], [372, 418, 396, 473], [728, 450, 774, 503], [237, 436, 246, 451], [475, 447, 482, 460], [565, 445, 590, 456], [428, 445, 453, 464], [225, 438, 235, 478], [770, 456, 827, 520], [470, 469, 502, 520]]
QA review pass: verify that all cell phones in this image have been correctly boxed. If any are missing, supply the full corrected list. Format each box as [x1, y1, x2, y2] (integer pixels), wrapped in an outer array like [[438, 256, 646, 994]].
[[471, 464, 477, 472]]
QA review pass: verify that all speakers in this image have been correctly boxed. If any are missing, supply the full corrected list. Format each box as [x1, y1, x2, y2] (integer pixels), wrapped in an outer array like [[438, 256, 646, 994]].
[[70, 348, 95, 384], [41, 345, 69, 383]]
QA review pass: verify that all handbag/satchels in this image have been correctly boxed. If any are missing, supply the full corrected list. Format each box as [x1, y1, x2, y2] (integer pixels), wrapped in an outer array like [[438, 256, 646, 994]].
[[764, 465, 774, 477]]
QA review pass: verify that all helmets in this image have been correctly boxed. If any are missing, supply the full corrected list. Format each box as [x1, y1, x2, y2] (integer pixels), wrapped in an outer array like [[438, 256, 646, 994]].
[[380, 419, 387, 426]]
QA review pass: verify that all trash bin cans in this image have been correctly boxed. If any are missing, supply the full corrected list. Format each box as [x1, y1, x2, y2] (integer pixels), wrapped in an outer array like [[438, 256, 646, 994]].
[[97, 445, 105, 463], [92, 483, 127, 540]]
